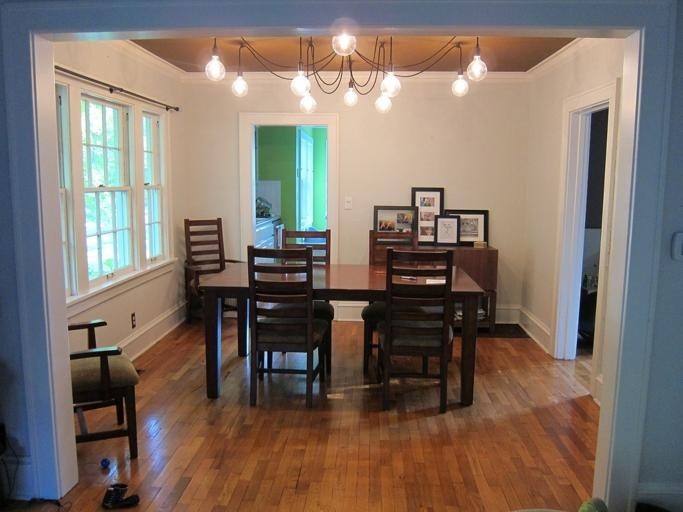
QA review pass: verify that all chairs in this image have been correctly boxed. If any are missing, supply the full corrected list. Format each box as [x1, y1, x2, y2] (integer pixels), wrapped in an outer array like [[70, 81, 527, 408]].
[[67, 319, 139, 458]]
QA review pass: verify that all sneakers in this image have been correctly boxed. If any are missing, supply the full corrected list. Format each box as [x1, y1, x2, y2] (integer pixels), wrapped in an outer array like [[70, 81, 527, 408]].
[[102, 483, 128, 508]]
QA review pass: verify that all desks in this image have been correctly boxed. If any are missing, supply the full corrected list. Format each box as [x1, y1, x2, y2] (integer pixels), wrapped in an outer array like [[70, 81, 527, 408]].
[[196, 266, 486, 408]]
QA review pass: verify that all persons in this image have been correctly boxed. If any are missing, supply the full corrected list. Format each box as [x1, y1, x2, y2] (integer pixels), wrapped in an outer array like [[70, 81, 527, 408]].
[[420, 226, 433, 235], [419, 213, 431, 221], [380, 222, 394, 230], [400, 216, 410, 223], [423, 199, 431, 206]]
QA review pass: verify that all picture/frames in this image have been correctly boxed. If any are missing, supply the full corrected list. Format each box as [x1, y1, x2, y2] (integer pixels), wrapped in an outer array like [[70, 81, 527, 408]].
[[373, 187, 489, 248]]
